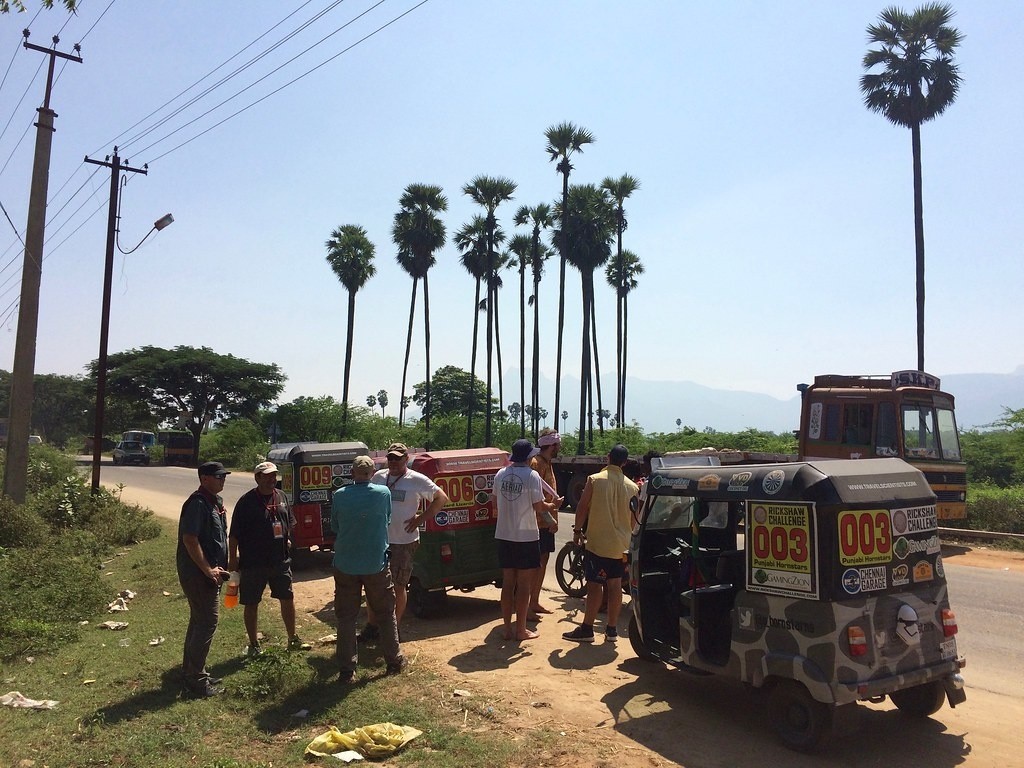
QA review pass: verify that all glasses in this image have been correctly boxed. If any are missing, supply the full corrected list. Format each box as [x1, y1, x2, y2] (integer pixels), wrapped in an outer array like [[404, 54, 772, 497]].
[[206, 474, 226, 479], [386, 456, 405, 461]]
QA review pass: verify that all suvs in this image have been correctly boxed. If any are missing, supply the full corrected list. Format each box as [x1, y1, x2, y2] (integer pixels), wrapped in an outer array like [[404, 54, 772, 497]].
[[113, 440, 150, 466]]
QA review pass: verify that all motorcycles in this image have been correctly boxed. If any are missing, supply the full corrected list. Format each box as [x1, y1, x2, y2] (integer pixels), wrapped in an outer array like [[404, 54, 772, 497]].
[[266, 440, 370, 572], [622, 455, 967, 756], [372, 446, 512, 620]]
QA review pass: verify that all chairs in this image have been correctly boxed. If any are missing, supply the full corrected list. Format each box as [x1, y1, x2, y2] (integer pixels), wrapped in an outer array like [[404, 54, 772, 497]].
[[716, 549, 745, 583]]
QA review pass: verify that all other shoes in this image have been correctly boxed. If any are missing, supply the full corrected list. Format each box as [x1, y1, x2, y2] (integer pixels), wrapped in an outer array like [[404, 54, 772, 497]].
[[288, 636, 312, 652], [358, 629, 378, 642], [248, 642, 263, 660], [208, 677, 223, 686], [184, 683, 218, 698]]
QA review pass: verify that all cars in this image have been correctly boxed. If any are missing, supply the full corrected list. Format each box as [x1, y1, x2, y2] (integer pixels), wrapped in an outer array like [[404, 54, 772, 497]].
[[29, 436, 43, 445]]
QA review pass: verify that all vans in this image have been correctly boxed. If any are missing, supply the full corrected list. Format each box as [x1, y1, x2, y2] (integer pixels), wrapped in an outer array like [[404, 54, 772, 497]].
[[162, 435, 198, 466], [120, 430, 155, 447]]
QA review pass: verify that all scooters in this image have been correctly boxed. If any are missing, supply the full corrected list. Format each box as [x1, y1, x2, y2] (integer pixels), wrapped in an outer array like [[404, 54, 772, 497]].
[[555, 521, 633, 598]]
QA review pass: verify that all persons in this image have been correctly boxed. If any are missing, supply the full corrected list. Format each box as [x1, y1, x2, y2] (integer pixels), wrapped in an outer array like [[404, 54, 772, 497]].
[[640, 450, 683, 531], [527, 430, 561, 620], [357, 443, 448, 644], [491, 439, 558, 642], [229, 462, 311, 656], [562, 444, 639, 642], [177, 461, 230, 697], [330, 456, 408, 680]]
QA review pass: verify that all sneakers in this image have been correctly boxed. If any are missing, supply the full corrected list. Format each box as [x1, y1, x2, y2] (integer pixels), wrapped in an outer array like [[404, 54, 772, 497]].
[[562, 627, 594, 642], [604, 628, 618, 641]]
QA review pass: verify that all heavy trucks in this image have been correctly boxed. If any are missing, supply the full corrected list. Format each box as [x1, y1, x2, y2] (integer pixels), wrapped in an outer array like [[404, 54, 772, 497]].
[[550, 369, 968, 532]]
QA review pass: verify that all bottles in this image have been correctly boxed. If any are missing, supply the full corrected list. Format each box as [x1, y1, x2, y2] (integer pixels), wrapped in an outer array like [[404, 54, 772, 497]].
[[224, 571, 239, 608]]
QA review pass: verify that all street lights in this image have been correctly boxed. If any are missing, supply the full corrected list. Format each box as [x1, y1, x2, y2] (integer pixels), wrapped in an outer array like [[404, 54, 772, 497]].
[[89, 211, 176, 499]]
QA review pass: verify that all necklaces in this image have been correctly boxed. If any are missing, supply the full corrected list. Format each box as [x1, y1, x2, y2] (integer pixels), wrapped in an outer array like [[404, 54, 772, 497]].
[[386, 468, 406, 488]]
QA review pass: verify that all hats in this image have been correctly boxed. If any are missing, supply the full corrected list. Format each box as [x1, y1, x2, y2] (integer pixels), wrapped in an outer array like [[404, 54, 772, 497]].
[[198, 462, 231, 475], [386, 443, 408, 456], [508, 439, 541, 462], [255, 462, 279, 474], [353, 456, 374, 474]]
